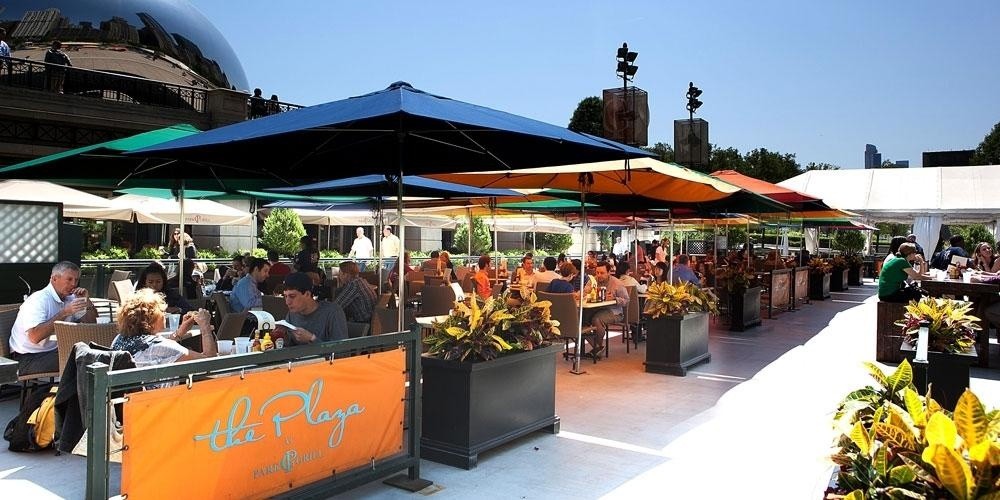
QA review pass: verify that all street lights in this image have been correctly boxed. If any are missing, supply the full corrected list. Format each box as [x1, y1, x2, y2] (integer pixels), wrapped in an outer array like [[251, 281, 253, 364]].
[[683, 84, 708, 165], [617, 42, 638, 144]]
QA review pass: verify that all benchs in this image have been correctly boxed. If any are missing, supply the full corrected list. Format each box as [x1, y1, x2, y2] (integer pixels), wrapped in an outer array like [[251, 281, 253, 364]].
[[876, 298, 910, 362]]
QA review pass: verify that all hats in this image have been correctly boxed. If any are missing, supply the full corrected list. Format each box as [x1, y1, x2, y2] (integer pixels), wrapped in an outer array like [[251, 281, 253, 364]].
[[284, 273, 313, 289], [950, 234, 964, 244], [340, 261, 359, 274]]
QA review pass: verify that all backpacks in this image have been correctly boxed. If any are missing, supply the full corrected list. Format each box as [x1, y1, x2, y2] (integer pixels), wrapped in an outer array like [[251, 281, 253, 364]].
[[4, 383, 59, 453]]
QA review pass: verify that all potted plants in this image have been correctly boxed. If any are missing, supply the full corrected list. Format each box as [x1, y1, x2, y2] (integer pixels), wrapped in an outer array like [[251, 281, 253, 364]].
[[891, 294, 982, 411], [806, 251, 866, 300], [824, 357, 1000, 500], [416, 279, 565, 470]]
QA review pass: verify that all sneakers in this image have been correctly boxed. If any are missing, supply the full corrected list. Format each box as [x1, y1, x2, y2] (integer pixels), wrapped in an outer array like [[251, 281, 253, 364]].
[[589, 346, 605, 357]]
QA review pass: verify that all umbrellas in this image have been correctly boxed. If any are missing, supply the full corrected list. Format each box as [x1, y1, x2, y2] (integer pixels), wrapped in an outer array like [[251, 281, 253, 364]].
[[0, 118, 375, 299], [122, 79, 662, 333]]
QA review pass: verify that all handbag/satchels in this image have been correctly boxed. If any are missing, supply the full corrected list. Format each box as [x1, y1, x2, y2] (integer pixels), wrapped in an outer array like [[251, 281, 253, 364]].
[[240, 311, 276, 341], [205, 299, 222, 336]]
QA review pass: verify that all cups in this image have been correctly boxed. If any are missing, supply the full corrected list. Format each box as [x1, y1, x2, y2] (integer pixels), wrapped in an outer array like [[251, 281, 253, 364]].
[[216, 340, 234, 356], [73, 297, 88, 315], [168, 313, 181, 331], [937, 271, 945, 281], [234, 337, 250, 354], [963, 273, 971, 283], [929, 269, 937, 276]]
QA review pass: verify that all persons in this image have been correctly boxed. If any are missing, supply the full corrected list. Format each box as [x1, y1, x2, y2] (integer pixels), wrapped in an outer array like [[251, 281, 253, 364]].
[[44, 41, 72, 95], [109, 287, 217, 390], [168, 228, 181, 284], [0, 27, 14, 75], [136, 261, 199, 325], [381, 225, 401, 272], [348, 226, 373, 273], [169, 232, 999, 371], [7, 258, 101, 377]]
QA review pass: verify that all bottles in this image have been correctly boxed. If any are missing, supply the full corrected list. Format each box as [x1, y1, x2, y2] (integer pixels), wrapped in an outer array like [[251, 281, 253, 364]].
[[262, 332, 274, 352], [591, 286, 607, 303], [251, 330, 261, 353]]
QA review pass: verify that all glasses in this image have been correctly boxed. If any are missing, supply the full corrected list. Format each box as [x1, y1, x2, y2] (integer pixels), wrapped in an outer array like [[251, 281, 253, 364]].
[[174, 233, 180, 235]]
